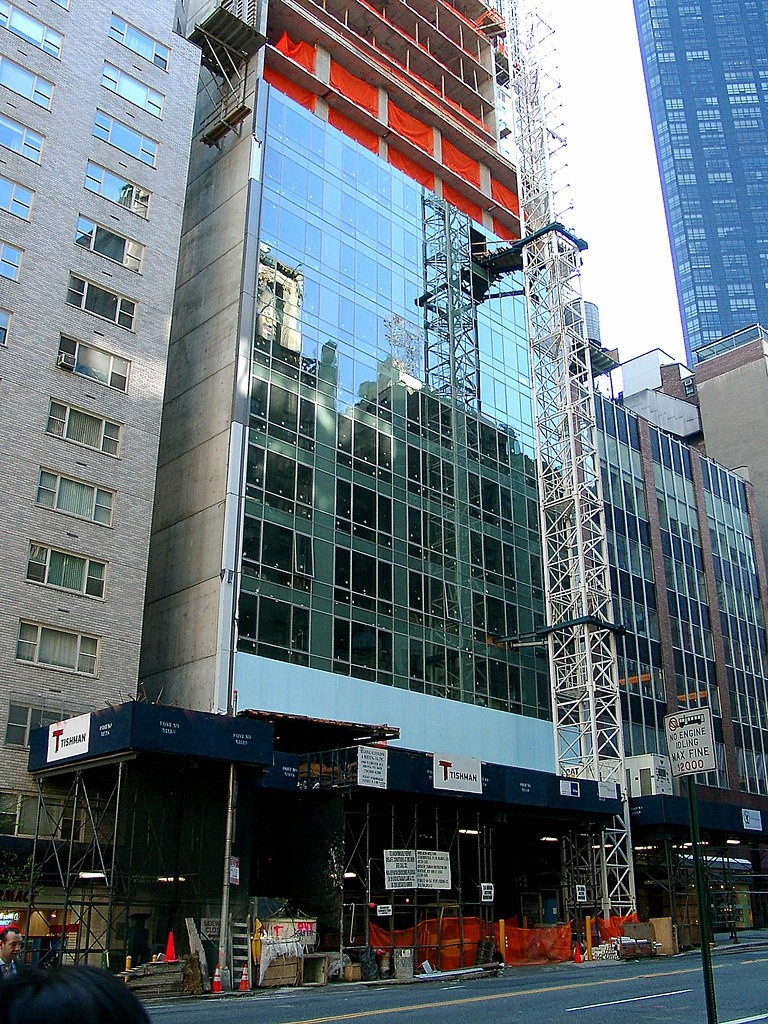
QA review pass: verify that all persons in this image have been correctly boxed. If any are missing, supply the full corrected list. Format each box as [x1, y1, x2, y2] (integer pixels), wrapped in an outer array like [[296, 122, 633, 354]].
[[1, 926, 29, 979], [1, 965, 151, 1024]]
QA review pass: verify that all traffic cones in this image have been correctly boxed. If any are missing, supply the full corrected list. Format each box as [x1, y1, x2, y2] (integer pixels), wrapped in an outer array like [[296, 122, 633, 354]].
[[236, 963, 252, 992], [573, 941, 583, 963], [209, 964, 225, 994], [161, 930, 181, 962]]
